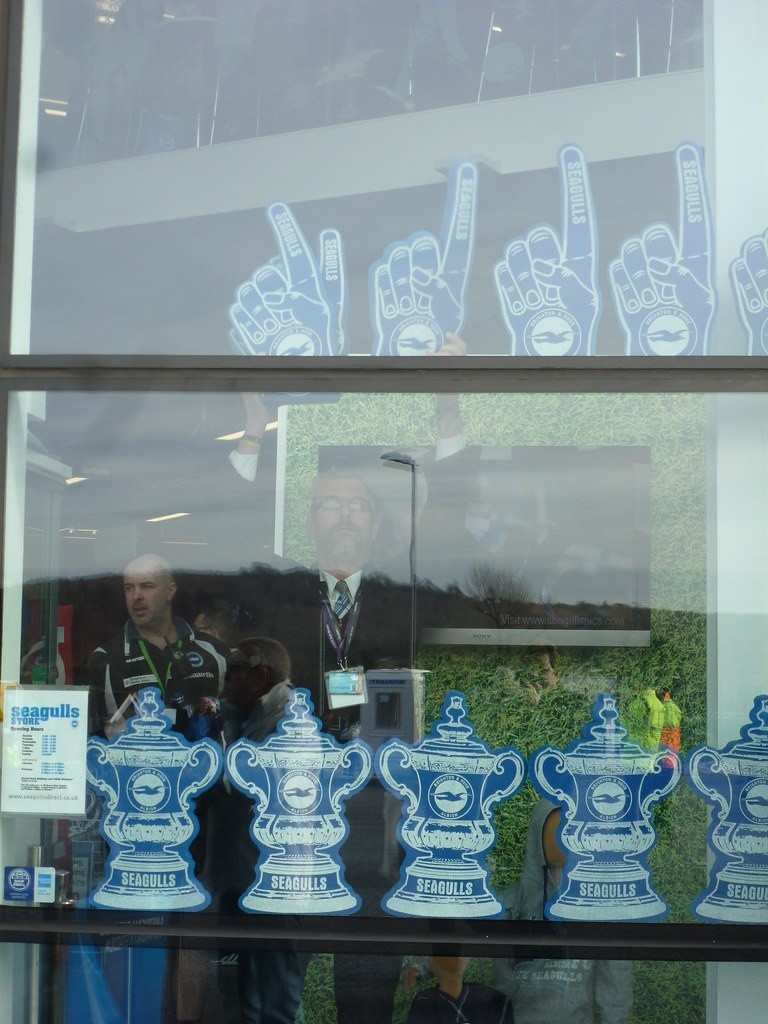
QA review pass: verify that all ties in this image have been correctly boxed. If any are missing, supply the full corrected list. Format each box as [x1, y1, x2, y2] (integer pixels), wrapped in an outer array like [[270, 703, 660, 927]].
[[333, 582, 352, 618]]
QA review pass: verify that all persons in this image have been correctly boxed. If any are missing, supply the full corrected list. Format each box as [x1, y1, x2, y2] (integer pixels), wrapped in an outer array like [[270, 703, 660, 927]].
[[404, 956, 516, 1024], [79, 554, 241, 880], [519, 644, 560, 708], [200, 637, 322, 1024], [189, 596, 239, 879], [620, 683, 668, 825], [191, 332, 468, 1024], [654, 686, 682, 826], [494, 797, 634, 1024]]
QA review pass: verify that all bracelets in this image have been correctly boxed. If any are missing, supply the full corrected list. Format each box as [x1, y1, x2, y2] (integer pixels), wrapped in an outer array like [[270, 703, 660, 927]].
[[434, 405, 459, 415], [241, 435, 263, 445]]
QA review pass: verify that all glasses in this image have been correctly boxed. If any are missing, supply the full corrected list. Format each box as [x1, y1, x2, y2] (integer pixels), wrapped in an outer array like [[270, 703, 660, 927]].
[[311, 496, 375, 520]]
